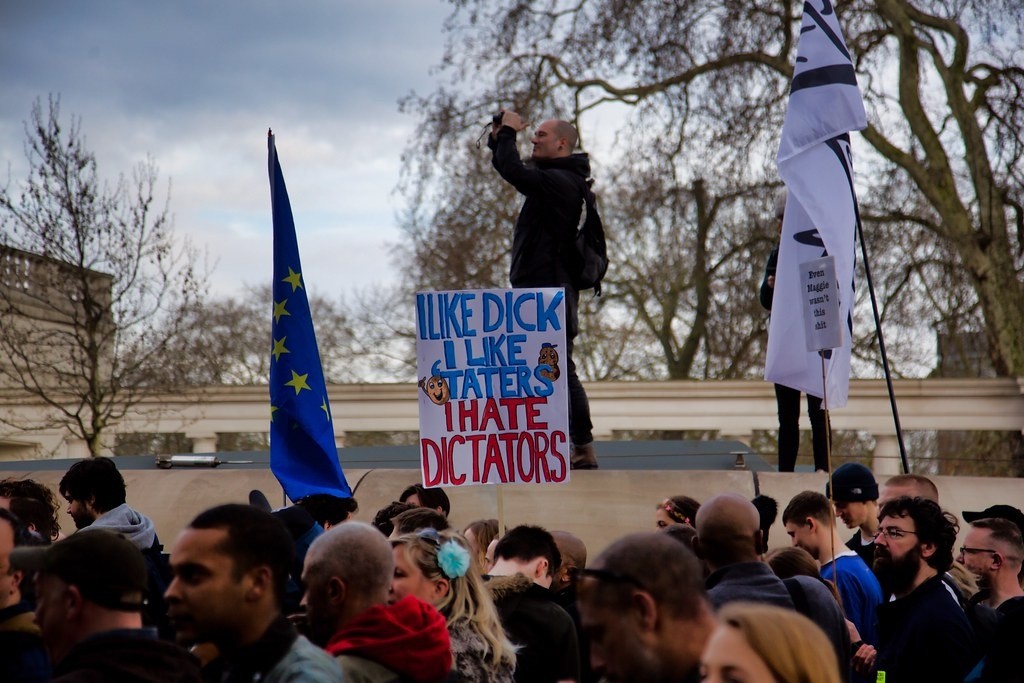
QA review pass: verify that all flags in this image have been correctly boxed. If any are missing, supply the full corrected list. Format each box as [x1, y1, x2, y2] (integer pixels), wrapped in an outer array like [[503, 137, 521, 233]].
[[763, 0, 868, 410], [269, 134, 353, 504]]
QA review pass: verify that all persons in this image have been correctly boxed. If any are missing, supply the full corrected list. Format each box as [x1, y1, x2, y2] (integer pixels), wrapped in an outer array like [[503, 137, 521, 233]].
[[486, 111, 608, 470], [0, 457, 1024, 682], [759, 187, 832, 474]]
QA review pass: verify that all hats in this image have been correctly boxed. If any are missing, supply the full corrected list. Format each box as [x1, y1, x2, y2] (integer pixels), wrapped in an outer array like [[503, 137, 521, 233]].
[[9, 529, 154, 612], [826, 463, 879, 501], [962, 504, 1024, 527]]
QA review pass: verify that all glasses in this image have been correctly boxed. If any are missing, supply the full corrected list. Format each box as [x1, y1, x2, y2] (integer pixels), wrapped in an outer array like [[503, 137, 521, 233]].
[[566, 567, 642, 599], [959, 548, 996, 559], [871, 527, 916, 538]]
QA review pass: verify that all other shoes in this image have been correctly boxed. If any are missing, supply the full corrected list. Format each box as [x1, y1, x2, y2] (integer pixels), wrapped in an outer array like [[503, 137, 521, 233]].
[[571, 447, 598, 469]]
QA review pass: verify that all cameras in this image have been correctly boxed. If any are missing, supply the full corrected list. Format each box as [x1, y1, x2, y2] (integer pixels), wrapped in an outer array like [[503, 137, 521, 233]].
[[491, 111, 525, 129]]
